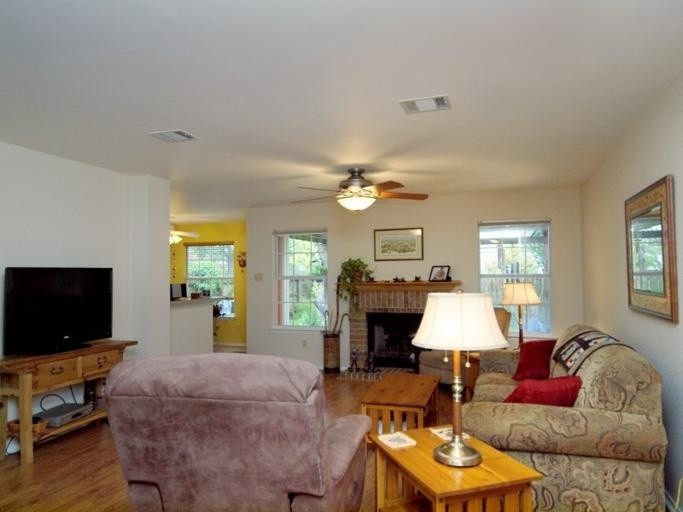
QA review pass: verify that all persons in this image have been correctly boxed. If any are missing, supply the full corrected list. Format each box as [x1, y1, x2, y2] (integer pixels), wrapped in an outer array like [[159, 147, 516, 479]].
[[431, 265, 446, 280]]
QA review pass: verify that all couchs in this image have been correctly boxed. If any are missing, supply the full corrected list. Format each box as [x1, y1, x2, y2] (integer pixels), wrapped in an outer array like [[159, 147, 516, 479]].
[[462, 324, 668, 512]]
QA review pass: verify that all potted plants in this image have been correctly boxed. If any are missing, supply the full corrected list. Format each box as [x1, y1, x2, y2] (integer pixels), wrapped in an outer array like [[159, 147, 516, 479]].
[[336, 259, 375, 312]]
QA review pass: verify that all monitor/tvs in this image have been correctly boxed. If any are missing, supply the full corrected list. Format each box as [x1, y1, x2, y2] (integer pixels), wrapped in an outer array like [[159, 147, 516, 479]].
[[2, 266, 113, 358]]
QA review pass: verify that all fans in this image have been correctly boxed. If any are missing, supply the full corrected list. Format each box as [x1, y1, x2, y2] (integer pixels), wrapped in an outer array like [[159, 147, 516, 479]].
[[170, 224, 199, 237], [292, 170, 429, 202]]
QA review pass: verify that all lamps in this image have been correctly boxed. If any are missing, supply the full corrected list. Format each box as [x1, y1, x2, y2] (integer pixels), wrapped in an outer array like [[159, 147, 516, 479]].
[[500, 283, 543, 349], [170, 234, 182, 244], [337, 194, 375, 210]]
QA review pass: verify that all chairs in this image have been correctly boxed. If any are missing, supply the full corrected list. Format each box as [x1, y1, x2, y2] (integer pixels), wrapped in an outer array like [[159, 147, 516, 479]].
[[418, 308, 511, 386], [101, 353, 372, 512]]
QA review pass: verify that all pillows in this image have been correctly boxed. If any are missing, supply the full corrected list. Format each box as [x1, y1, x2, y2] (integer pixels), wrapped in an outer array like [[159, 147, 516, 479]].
[[512, 339, 556, 381], [504, 377, 582, 407]]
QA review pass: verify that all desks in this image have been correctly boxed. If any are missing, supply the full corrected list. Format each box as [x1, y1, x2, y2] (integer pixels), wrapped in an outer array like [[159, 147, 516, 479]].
[[0, 340, 137, 467]]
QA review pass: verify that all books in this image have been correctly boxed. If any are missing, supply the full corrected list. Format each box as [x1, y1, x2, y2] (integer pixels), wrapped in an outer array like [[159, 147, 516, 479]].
[[430, 426, 470, 442], [375, 431, 417, 449]]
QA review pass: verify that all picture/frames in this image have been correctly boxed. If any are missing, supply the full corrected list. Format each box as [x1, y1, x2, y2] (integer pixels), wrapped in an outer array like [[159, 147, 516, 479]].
[[429, 265, 450, 281], [625, 174, 679, 323], [374, 227, 423, 261]]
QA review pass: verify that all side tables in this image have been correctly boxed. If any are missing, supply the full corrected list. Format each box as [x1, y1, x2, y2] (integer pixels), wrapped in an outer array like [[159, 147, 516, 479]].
[[462, 352, 479, 402], [368, 425, 543, 512]]
